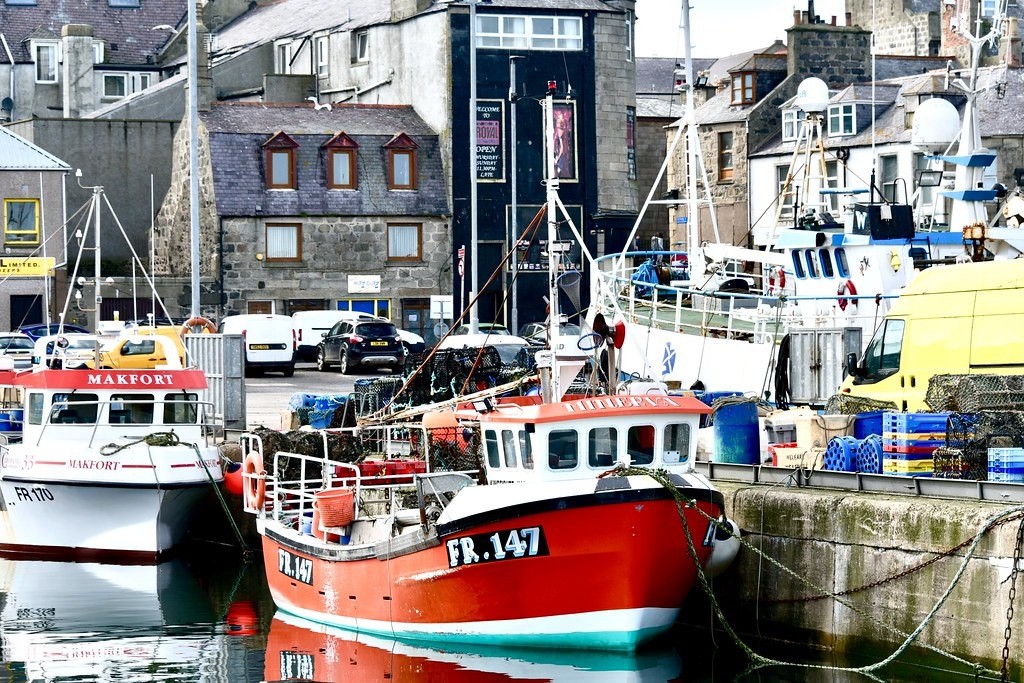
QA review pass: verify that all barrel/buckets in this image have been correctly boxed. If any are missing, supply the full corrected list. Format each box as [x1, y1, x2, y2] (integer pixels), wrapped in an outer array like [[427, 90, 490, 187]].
[[314, 488, 354, 527], [714, 402, 760, 466], [0, 410, 23, 432], [855, 410, 888, 438], [311, 501, 342, 541], [302, 512, 315, 533], [338, 536, 351, 545]]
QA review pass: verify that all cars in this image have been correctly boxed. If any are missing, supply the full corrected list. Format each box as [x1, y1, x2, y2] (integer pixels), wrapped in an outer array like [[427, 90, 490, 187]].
[[65, 333, 107, 368], [519, 321, 580, 348], [439, 333, 531, 372], [0, 332, 35, 364], [455, 323, 511, 335], [17, 323, 91, 343]]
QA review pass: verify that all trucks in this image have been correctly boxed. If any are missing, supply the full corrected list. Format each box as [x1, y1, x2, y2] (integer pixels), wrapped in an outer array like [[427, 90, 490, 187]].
[[292, 311, 425, 362], [836, 258, 1024, 414]]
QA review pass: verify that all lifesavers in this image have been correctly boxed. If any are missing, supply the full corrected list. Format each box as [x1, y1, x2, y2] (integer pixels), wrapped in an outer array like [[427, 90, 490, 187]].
[[241, 451, 267, 512], [835, 278, 859, 313], [768, 265, 786, 297], [178, 315, 218, 343]]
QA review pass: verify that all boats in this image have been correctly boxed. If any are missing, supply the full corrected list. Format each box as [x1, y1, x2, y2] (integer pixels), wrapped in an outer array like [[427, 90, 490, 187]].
[[0, 561, 217, 683], [263, 609, 682, 683], [583, 0, 1024, 417], [235, 94, 726, 653], [0, 169, 224, 566]]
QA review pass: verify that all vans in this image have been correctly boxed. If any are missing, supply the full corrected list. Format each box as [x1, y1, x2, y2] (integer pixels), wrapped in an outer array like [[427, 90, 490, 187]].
[[218, 315, 296, 377], [65, 325, 192, 370]]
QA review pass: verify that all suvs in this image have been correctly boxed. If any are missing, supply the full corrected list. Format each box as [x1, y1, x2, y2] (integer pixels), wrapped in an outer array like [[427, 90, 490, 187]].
[[317, 315, 405, 375]]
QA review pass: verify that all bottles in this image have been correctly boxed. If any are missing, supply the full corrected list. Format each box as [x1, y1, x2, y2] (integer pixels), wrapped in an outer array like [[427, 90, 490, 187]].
[[706, 516, 741, 569]]
